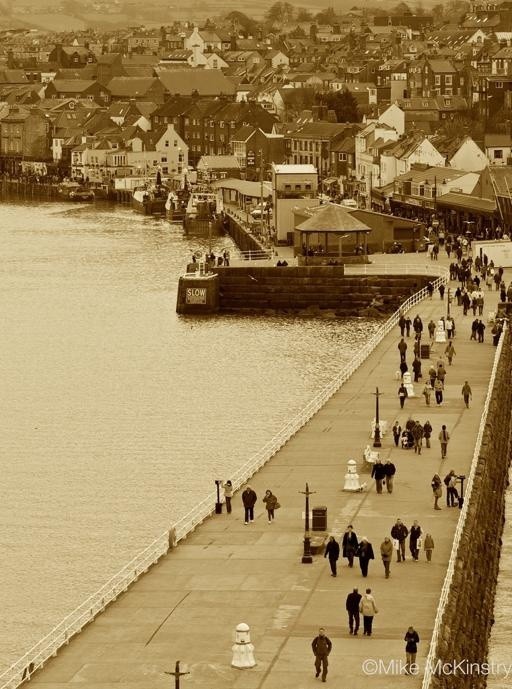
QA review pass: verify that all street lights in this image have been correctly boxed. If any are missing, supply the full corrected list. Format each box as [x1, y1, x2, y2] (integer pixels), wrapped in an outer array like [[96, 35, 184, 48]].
[[298, 482, 317, 563], [361, 172, 383, 211], [372, 387, 384, 447], [434, 174, 447, 220], [445, 288, 455, 320]]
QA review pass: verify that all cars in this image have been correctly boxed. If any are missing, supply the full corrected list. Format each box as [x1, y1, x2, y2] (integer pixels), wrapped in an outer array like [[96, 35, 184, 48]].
[[342, 199, 357, 208], [250, 203, 271, 218]]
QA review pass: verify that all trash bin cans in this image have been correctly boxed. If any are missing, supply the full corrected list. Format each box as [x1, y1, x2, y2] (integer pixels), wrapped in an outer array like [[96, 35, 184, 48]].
[[420, 344, 429, 358], [312, 506, 327, 530]]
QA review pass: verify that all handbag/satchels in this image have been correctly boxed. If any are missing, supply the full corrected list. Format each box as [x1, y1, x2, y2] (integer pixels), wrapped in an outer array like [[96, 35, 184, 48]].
[[274, 501, 282, 510]]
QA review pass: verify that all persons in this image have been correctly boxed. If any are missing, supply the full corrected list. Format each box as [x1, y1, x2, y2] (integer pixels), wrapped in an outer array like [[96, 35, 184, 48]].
[[380, 535, 393, 579], [369, 206, 512, 263], [273, 239, 370, 267], [220, 480, 232, 515], [382, 459, 396, 496], [311, 627, 332, 682], [358, 587, 379, 637], [345, 586, 363, 636], [444, 470, 457, 507], [262, 489, 278, 524], [396, 263, 511, 410], [422, 532, 435, 563], [404, 519, 424, 562], [390, 518, 409, 563], [447, 473, 459, 499], [341, 524, 358, 568], [404, 626, 420, 675], [354, 536, 375, 578], [371, 458, 384, 496], [323, 535, 339, 577], [391, 415, 433, 456], [191, 249, 231, 268], [241, 484, 257, 525], [438, 424, 450, 459], [429, 473, 443, 510]]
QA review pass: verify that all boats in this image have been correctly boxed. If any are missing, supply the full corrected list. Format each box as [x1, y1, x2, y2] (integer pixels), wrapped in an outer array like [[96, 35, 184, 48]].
[[132, 167, 231, 240], [70, 188, 94, 204]]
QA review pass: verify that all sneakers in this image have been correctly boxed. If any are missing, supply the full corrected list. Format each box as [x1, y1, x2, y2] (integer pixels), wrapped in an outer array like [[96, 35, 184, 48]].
[[316, 670, 321, 678], [348, 563, 353, 567], [367, 633, 372, 636], [397, 559, 432, 563], [441, 454, 446, 458], [426, 404, 442, 407], [364, 632, 366, 635], [405, 670, 414, 675], [354, 631, 358, 635], [268, 521, 271, 524], [244, 522, 247, 524], [322, 679, 326, 682], [250, 520, 254, 523], [331, 573, 337, 577], [395, 444, 430, 454], [448, 504, 457, 507], [349, 631, 353, 634], [385, 571, 391, 578], [377, 491, 391, 495], [363, 574, 367, 577], [434, 506, 441, 510]]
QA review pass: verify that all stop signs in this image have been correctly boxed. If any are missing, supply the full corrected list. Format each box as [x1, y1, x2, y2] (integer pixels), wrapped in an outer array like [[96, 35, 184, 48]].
[[433, 220, 439, 227]]
